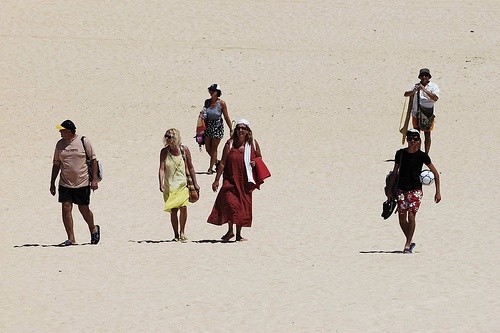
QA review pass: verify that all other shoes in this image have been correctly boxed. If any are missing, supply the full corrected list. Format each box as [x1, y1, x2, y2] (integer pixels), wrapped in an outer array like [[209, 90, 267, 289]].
[[180, 236, 188, 241], [236, 236, 248, 241], [215, 160, 220, 172], [221, 234, 234, 241], [172, 236, 179, 241], [207, 169, 213, 174]]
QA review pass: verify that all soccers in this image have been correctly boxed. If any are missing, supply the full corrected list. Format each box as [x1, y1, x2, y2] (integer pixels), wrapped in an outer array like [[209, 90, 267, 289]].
[[420, 169, 435, 185]]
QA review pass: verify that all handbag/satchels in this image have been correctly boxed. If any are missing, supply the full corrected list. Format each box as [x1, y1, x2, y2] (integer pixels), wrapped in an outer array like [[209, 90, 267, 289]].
[[88, 160, 102, 182], [186, 168, 199, 202], [384, 171, 399, 200], [416, 103, 435, 131]]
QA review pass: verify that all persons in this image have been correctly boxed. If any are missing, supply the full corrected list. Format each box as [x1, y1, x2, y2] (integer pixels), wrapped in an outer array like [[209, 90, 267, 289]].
[[203, 83, 233, 175], [387, 129, 441, 254], [404, 68, 440, 155], [206, 119, 271, 242], [159, 128, 200, 242], [50, 120, 100, 247]]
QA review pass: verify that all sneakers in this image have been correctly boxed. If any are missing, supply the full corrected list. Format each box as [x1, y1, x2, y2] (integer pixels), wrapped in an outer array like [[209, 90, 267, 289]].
[[384, 201, 396, 220], [381, 200, 389, 217]]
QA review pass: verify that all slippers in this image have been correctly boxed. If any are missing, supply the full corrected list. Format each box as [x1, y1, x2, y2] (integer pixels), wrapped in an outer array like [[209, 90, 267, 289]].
[[410, 243, 416, 252], [91, 225, 100, 244], [61, 240, 78, 246], [403, 248, 410, 254]]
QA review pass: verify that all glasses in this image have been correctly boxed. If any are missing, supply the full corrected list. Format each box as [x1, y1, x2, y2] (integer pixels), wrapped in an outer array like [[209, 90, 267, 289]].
[[236, 127, 247, 130], [164, 134, 173, 139], [407, 137, 420, 141]]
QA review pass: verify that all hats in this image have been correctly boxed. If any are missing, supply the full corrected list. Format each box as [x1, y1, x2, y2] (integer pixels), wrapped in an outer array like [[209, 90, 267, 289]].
[[418, 69, 431, 79], [54, 120, 76, 130], [208, 83, 221, 91], [406, 128, 420, 136]]
